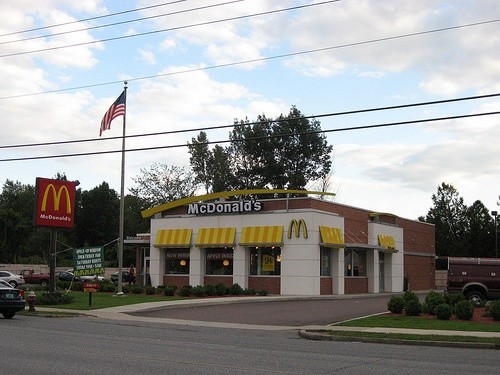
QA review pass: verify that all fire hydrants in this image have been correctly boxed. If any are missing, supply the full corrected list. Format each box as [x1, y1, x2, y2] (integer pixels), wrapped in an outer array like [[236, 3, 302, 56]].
[[27, 291, 36, 312]]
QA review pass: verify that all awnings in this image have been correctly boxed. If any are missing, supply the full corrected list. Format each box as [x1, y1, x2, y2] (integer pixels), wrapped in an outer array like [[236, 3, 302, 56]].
[[195, 227, 236, 248], [319, 225, 345, 249], [238, 225, 283, 247], [378, 234, 399, 254], [152, 229, 193, 249]]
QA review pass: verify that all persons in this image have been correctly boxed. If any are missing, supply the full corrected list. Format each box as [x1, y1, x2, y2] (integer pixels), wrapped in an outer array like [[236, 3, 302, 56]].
[[127, 264, 136, 286]]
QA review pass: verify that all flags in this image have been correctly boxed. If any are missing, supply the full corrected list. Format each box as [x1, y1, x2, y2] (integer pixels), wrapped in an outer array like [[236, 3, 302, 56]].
[[99, 89, 126, 136]]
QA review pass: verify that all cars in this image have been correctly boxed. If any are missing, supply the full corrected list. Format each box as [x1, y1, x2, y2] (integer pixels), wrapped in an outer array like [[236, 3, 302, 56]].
[[0, 270, 26, 288], [67, 269, 105, 282], [111, 271, 136, 282], [0, 282, 26, 318], [55, 271, 80, 282]]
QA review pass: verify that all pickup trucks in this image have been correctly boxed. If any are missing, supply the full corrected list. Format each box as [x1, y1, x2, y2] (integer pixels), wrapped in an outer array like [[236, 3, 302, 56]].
[[19, 269, 58, 286]]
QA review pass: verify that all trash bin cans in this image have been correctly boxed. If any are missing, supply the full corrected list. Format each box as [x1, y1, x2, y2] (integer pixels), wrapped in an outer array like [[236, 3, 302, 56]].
[[140, 273, 150, 286]]
